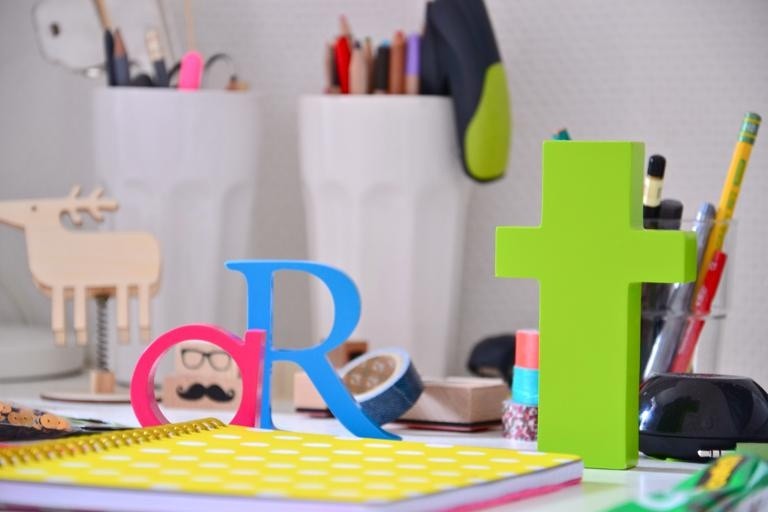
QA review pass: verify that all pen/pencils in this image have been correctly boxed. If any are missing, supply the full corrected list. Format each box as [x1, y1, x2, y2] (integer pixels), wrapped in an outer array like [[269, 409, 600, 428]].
[[94, 1, 246, 90], [324, 14, 419, 95], [550, 111, 761, 380]]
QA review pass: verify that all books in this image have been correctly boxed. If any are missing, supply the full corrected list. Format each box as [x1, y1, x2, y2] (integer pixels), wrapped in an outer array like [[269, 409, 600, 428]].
[[0, 418, 584, 511], [291, 372, 513, 430], [603, 440, 767, 511]]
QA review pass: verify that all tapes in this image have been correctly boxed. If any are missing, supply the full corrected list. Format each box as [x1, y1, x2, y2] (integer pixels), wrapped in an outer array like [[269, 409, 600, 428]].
[[337, 345, 424, 426]]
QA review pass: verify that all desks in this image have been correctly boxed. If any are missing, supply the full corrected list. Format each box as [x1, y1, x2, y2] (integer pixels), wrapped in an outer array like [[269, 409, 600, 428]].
[[0, 362, 768, 512]]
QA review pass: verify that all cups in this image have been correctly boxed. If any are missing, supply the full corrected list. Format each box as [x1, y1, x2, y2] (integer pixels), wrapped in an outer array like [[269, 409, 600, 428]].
[[639, 307, 727, 387]]
